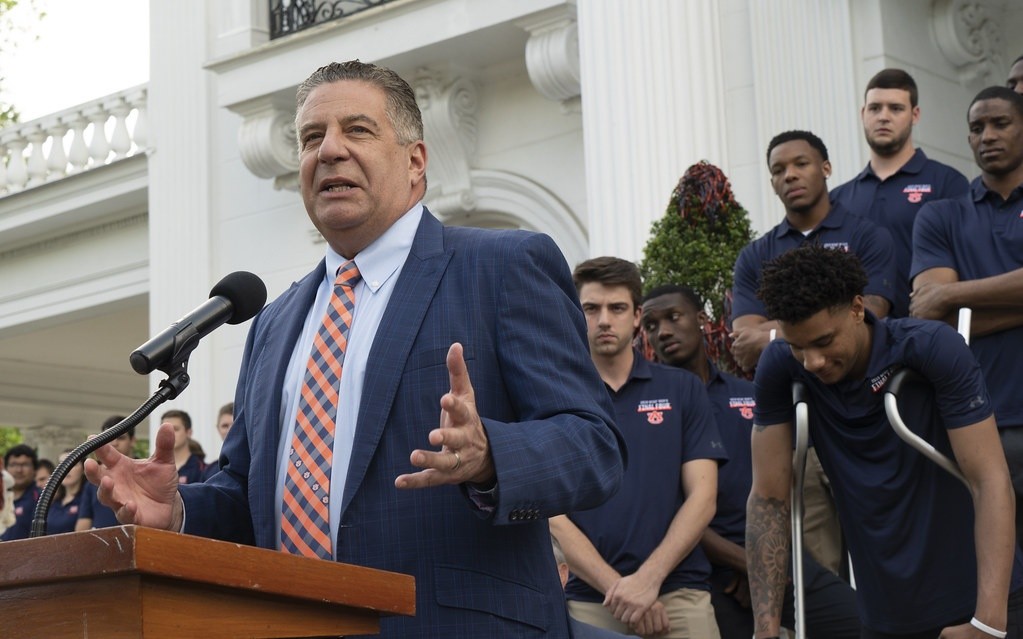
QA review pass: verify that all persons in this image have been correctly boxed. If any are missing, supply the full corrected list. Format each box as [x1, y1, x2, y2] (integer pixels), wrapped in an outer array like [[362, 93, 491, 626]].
[[907, 85, 1023, 639], [74, 416, 139, 532], [745, 246, 1023, 639], [201, 403, 234, 484], [545, 256, 731, 639], [728, 130, 898, 372], [1006, 55, 1023, 96], [829, 67, 969, 319], [640, 285, 862, 639], [0, 444, 87, 541], [83, 60, 630, 639], [161, 410, 207, 485]]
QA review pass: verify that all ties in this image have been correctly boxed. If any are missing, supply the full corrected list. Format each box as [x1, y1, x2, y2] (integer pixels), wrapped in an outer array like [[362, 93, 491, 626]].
[[281, 259, 362, 560]]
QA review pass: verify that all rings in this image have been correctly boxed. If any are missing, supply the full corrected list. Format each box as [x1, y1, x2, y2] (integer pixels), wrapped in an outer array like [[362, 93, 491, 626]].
[[449, 453, 461, 471]]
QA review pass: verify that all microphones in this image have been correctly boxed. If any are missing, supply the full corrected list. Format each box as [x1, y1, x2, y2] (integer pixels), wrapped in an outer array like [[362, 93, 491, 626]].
[[130, 271, 267, 375]]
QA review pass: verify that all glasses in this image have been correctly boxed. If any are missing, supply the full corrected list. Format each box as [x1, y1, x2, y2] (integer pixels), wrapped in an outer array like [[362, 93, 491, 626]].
[[7, 461, 28, 467]]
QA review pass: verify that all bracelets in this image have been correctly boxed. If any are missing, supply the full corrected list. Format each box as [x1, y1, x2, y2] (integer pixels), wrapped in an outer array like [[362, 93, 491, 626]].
[[770, 329, 776, 342], [970, 617, 1007, 639]]
